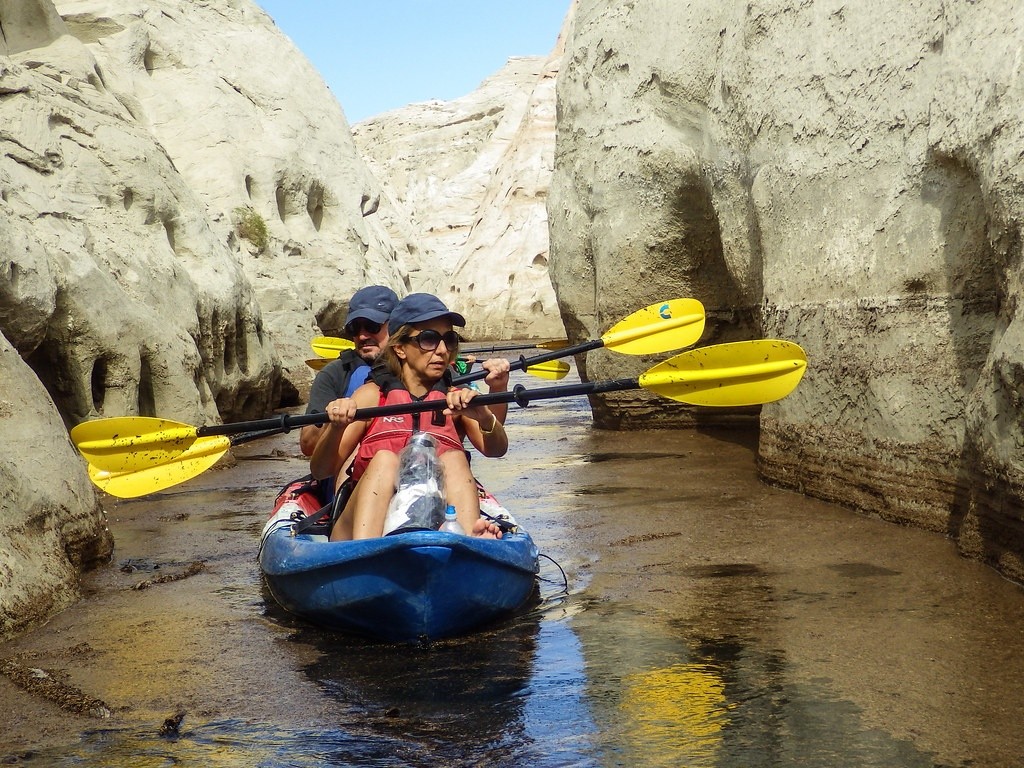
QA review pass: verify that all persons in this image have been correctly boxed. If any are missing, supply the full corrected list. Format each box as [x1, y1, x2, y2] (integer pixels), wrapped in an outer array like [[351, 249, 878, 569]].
[[450, 348, 477, 377], [298, 283, 509, 495], [308, 293, 509, 541]]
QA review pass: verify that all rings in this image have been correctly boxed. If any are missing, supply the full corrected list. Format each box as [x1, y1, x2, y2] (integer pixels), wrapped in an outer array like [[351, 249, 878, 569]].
[[331, 406, 340, 410]]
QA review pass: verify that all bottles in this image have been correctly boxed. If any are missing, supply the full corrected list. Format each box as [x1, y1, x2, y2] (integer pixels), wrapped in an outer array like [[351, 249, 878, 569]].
[[437, 506, 466, 535]]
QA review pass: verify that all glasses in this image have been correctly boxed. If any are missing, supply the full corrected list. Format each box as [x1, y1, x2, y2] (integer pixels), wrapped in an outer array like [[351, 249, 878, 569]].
[[345, 320, 383, 336], [407, 329, 459, 352]]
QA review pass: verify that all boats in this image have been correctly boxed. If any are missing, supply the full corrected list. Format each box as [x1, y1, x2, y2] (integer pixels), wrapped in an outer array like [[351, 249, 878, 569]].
[[255, 474, 566, 647]]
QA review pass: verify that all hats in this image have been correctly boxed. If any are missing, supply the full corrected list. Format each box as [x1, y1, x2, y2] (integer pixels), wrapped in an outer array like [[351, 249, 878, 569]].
[[388, 293, 466, 337], [345, 285, 398, 327]]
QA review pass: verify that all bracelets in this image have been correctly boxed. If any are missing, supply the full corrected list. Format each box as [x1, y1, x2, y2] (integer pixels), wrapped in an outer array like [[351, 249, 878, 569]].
[[478, 413, 496, 435]]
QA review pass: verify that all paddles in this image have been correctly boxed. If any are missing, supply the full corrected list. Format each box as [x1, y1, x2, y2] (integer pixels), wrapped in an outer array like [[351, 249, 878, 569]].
[[87, 296, 706, 499], [457, 358, 570, 381], [458, 339, 572, 354], [310, 337, 357, 358], [304, 358, 340, 372], [69, 340, 806, 471]]
